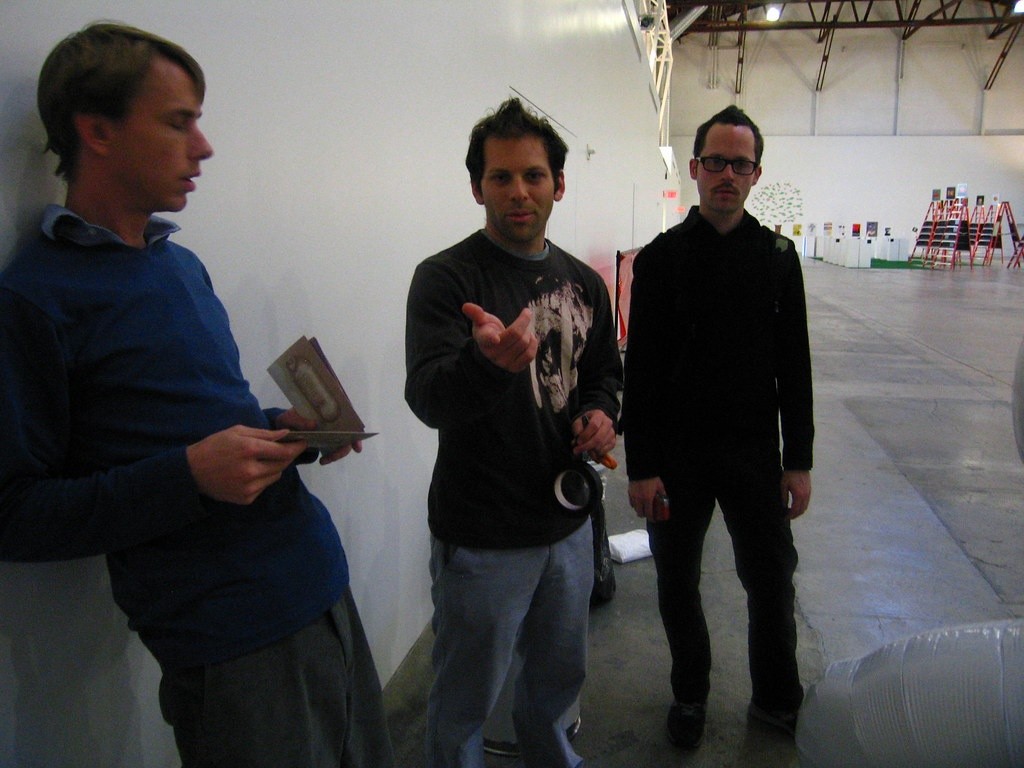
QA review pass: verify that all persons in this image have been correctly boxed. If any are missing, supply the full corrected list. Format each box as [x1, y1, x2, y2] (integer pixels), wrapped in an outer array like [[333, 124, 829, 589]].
[[620, 103, 816, 749], [403, 98, 624, 768], [0, 25, 401, 768]]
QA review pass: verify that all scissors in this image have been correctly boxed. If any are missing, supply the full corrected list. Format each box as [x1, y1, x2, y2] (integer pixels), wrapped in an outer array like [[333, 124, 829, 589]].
[[581, 414, 618, 471]]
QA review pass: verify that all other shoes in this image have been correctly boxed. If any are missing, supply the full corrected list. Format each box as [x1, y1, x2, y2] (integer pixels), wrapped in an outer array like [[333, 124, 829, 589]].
[[748, 700, 800, 736], [665, 700, 707, 751]]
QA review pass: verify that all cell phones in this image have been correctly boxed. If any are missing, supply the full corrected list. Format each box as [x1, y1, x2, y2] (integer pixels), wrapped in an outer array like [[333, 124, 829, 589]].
[[652, 495, 669, 521]]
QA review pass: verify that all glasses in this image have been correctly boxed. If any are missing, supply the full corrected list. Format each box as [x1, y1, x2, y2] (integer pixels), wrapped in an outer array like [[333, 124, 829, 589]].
[[695, 156, 758, 176]]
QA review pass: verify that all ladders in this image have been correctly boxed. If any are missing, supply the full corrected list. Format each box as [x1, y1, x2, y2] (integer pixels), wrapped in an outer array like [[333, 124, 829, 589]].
[[982, 201, 1024, 268], [908, 196, 996, 273]]
